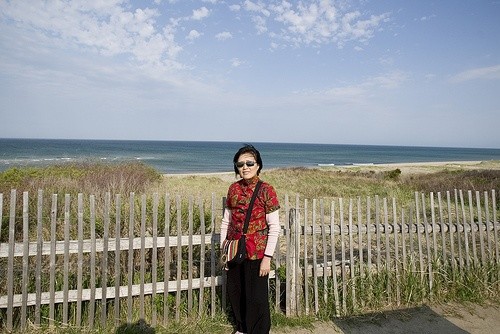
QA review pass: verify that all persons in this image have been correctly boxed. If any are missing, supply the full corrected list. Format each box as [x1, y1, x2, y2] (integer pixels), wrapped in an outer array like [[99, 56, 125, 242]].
[[219, 144, 281, 334]]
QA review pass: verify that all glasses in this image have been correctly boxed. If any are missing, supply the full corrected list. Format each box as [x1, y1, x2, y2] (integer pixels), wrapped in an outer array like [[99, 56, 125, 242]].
[[236, 161, 257, 167]]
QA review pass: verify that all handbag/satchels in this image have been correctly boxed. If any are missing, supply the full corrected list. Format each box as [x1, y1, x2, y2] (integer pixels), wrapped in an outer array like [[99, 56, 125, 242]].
[[221, 179, 263, 272]]
